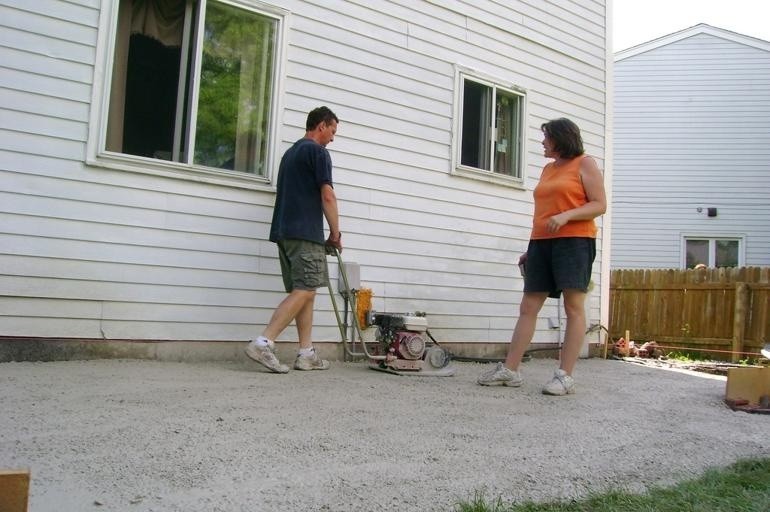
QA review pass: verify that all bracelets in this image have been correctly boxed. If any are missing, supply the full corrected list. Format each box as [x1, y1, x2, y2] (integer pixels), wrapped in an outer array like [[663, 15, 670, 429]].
[[330, 231, 342, 240]]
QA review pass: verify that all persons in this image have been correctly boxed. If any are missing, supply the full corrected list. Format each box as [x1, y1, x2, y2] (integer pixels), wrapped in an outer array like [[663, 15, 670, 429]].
[[242, 105, 346, 372], [474, 116, 608, 396]]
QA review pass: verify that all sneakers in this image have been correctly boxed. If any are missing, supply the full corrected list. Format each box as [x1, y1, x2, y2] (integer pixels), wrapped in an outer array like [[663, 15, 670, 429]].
[[477, 362, 524, 388], [243, 339, 291, 374], [293, 349, 331, 373], [542, 369, 577, 396]]
